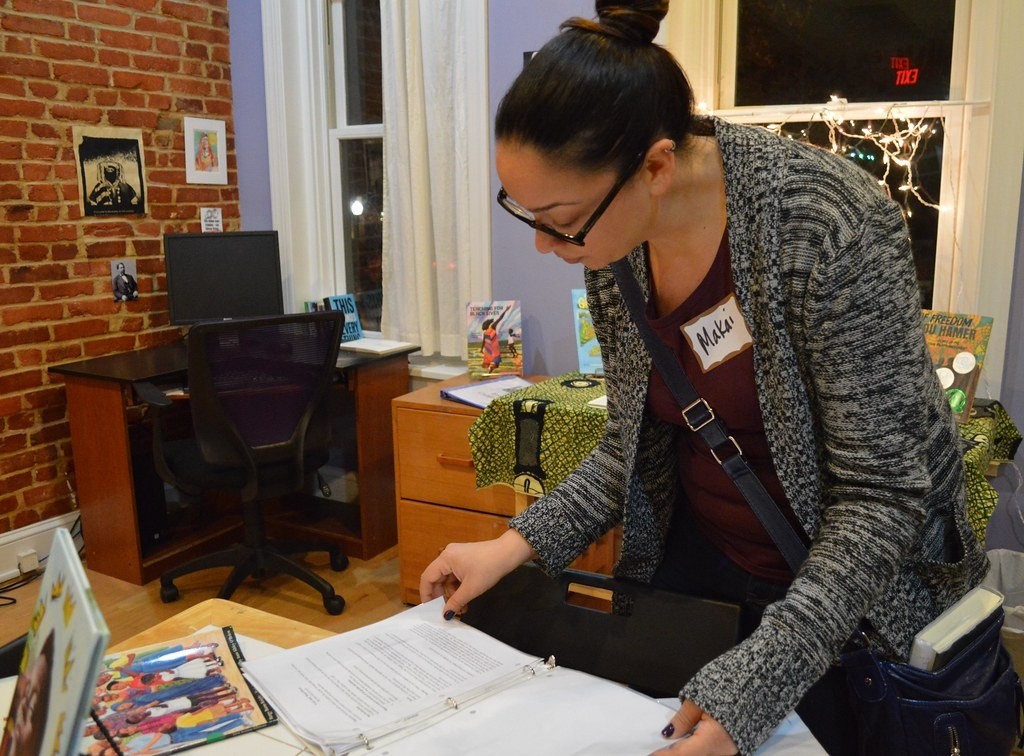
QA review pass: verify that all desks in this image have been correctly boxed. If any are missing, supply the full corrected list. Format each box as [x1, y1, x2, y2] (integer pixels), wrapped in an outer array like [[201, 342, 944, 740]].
[[468, 372, 1023, 600], [48, 339, 421, 585], [76, 599, 682, 756]]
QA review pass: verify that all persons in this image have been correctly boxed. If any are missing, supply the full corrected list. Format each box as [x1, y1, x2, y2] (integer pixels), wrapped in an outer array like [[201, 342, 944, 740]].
[[7, 629, 55, 755], [420, 0, 991, 755], [113, 263, 138, 301]]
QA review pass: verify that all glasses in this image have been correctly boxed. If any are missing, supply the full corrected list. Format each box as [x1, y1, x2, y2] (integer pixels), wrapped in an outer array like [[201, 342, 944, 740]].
[[497, 143, 647, 248]]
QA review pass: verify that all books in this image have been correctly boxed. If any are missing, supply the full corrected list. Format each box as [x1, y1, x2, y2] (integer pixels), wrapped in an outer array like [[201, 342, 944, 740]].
[[302, 294, 417, 355], [466, 300, 523, 381], [918, 310, 994, 424], [571, 286, 607, 376], [0, 528, 279, 756], [910, 583, 1004, 671]]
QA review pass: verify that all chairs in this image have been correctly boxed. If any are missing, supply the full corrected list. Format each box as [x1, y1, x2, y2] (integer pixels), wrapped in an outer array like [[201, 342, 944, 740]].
[[460, 563, 740, 699], [133, 310, 349, 615]]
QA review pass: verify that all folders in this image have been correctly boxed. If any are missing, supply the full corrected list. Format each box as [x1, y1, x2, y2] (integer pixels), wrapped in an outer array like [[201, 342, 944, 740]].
[[439, 375, 535, 409]]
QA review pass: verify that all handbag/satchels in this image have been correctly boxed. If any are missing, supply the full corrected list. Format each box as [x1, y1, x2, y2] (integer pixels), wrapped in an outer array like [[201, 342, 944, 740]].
[[846, 605, 1022, 756]]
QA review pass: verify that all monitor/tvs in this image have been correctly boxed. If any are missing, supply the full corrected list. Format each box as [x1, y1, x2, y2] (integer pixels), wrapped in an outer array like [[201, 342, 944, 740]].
[[163, 230, 284, 327]]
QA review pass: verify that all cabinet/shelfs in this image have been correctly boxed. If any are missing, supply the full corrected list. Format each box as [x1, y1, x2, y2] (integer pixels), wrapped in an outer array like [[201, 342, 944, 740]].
[[391, 373, 623, 612]]
